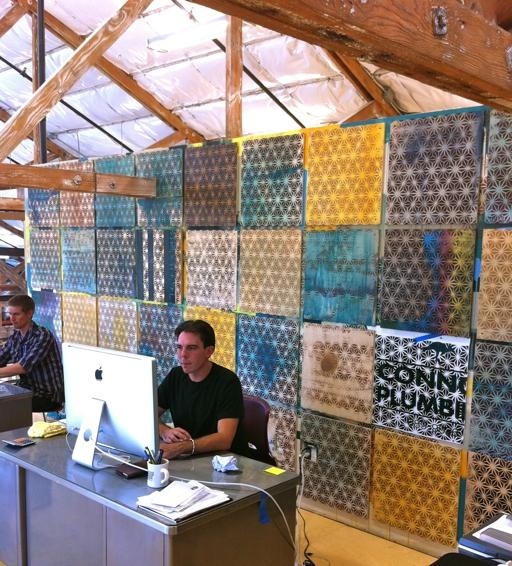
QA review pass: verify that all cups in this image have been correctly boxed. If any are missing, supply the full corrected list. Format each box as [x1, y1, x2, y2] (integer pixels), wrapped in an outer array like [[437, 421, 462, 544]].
[[146, 459, 169, 489]]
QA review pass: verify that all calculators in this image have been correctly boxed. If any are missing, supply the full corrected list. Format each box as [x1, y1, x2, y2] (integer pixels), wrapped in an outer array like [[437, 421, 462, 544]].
[[2, 437, 36, 448]]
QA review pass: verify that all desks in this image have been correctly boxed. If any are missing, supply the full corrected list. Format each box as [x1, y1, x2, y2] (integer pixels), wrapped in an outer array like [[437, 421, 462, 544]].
[[0, 424, 303, 566]]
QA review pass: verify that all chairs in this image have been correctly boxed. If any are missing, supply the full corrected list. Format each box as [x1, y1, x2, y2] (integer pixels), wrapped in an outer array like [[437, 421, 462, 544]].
[[231, 393, 279, 467]]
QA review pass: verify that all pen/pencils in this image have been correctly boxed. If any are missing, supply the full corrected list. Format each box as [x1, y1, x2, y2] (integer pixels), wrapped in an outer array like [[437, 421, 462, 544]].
[[144, 446, 164, 464]]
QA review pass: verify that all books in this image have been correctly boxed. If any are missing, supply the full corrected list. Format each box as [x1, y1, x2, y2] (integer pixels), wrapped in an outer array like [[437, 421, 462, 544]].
[[135, 479, 235, 524]]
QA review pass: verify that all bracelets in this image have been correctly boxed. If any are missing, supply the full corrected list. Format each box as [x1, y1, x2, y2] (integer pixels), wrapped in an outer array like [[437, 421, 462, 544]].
[[187, 438, 196, 456]]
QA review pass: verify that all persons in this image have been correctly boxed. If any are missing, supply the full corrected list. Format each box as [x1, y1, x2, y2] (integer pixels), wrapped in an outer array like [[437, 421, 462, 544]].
[[0, 294, 64, 414], [156, 320, 246, 460]]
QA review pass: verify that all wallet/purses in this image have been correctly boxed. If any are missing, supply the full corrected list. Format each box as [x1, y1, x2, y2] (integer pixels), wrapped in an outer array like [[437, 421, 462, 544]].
[[114, 461, 148, 480]]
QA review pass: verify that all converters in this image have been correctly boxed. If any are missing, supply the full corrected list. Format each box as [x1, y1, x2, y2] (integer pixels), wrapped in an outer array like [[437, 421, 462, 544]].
[[305, 560, 315, 566]]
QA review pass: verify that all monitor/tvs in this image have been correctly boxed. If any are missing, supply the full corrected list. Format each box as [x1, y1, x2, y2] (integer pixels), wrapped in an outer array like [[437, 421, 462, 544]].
[[61, 341, 159, 472]]
